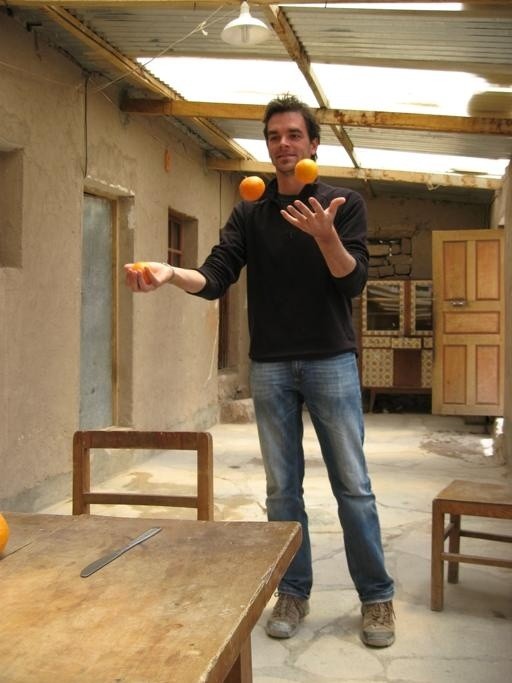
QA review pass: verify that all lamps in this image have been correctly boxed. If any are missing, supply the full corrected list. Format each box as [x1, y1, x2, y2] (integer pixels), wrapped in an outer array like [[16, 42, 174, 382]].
[[220, 0, 271, 48]]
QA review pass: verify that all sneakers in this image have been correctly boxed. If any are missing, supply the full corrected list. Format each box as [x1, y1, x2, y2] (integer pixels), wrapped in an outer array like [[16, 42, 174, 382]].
[[360, 600, 396, 647], [265, 590, 311, 639]]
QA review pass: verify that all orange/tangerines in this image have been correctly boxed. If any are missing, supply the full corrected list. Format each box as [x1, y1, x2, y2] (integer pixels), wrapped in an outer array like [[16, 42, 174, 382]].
[[130, 263, 151, 285], [294, 158, 320, 186], [0, 514, 10, 553], [238, 174, 266, 202]]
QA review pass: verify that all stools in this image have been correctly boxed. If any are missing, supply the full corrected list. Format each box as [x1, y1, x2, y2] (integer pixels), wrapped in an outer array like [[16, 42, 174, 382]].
[[432, 481, 512, 617]]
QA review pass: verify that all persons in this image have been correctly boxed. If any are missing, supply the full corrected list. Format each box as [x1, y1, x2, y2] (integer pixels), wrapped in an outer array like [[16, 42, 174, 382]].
[[123, 96, 397, 648]]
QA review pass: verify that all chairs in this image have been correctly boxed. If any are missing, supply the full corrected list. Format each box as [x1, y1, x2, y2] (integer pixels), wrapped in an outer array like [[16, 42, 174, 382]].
[[71, 428, 218, 525]]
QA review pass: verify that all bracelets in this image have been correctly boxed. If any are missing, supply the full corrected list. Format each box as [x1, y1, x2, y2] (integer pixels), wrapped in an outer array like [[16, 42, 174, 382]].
[[163, 263, 175, 282]]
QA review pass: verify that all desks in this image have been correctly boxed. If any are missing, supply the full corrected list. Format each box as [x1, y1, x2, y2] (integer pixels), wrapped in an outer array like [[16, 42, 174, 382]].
[[0, 513, 306, 682]]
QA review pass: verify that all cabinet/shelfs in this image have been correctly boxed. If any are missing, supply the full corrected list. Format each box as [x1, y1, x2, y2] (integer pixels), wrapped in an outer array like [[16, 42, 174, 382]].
[[360, 277, 435, 413]]
